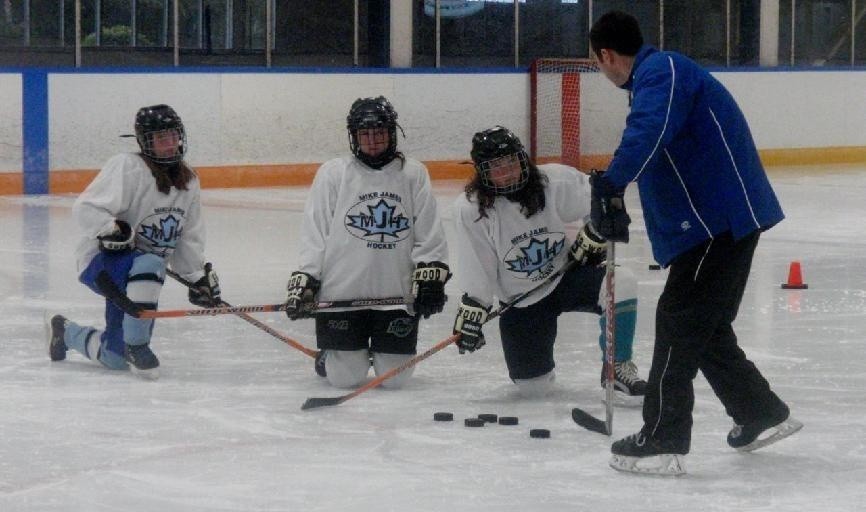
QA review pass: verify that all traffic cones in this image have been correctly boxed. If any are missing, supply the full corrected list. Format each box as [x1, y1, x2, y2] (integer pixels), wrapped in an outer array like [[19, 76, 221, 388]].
[[778, 259, 810, 291], [781, 262, 807, 289]]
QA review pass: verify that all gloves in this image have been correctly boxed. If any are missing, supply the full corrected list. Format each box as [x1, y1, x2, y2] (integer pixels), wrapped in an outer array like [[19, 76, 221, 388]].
[[588, 170, 632, 244], [189, 263, 221, 307], [286, 272, 320, 321], [568, 222, 606, 270], [412, 262, 449, 318], [100, 221, 136, 254], [453, 294, 492, 353]]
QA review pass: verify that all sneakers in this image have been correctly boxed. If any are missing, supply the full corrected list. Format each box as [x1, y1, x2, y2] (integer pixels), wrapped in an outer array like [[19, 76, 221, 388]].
[[601, 362, 647, 396], [50, 314, 67, 360], [728, 399, 789, 448], [124, 343, 159, 369], [612, 422, 691, 456], [315, 349, 328, 378]]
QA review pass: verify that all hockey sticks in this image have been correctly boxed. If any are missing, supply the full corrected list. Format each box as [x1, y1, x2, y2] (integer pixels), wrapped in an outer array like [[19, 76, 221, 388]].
[[93, 268, 450, 320], [300, 258, 581, 411], [571, 241, 617, 437]]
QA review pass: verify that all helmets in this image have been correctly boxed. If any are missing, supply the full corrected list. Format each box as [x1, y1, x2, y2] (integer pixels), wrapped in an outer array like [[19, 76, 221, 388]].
[[471, 126, 531, 195], [347, 96, 399, 168], [134, 104, 187, 165]]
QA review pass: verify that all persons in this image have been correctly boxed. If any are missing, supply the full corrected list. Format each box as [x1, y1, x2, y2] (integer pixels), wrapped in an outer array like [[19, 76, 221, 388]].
[[453, 124, 651, 398], [49, 104, 221, 371], [589, 10, 789, 458], [286, 94, 453, 390]]
